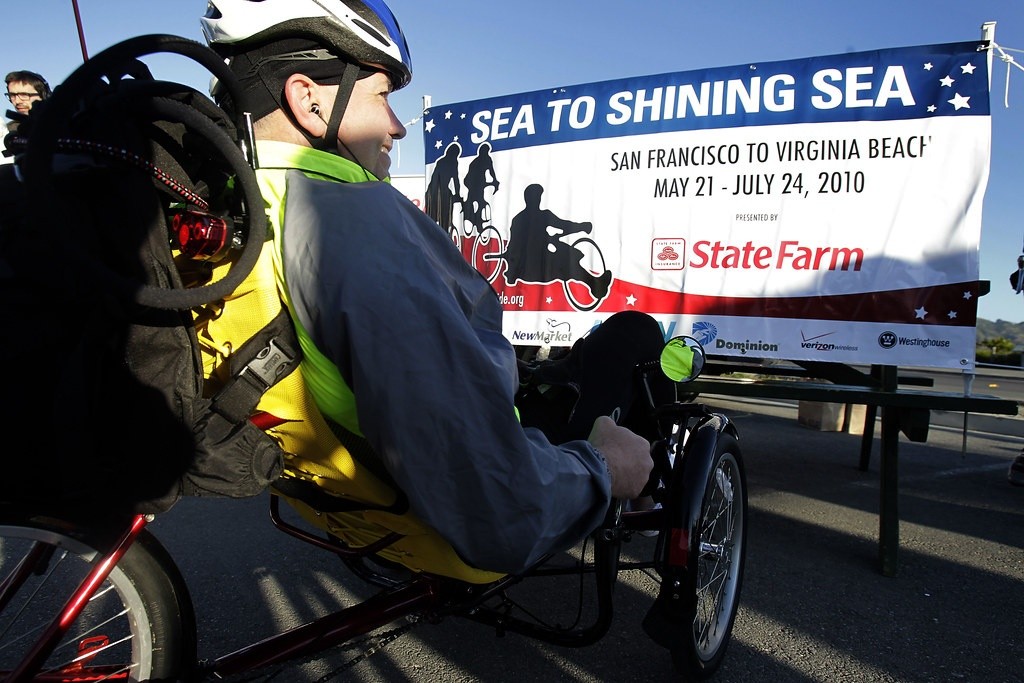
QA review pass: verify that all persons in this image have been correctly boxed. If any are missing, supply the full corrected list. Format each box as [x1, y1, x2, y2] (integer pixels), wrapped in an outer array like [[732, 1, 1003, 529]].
[[191, 0, 682, 579], [3, 70, 51, 130]]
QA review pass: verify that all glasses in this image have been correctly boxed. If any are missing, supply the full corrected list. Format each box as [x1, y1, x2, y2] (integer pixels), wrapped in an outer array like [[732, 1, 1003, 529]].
[[4, 92, 39, 100]]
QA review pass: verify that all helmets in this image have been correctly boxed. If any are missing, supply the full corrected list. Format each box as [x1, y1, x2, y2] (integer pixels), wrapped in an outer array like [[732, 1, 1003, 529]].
[[200, 1, 413, 92]]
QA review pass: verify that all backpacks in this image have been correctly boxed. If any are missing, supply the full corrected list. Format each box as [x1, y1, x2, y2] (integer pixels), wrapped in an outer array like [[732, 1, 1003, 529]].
[[1, 150, 288, 522]]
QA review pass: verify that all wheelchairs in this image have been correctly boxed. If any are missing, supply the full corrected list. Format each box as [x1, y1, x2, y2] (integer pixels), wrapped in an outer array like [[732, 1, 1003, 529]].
[[0, 39, 749, 683]]
